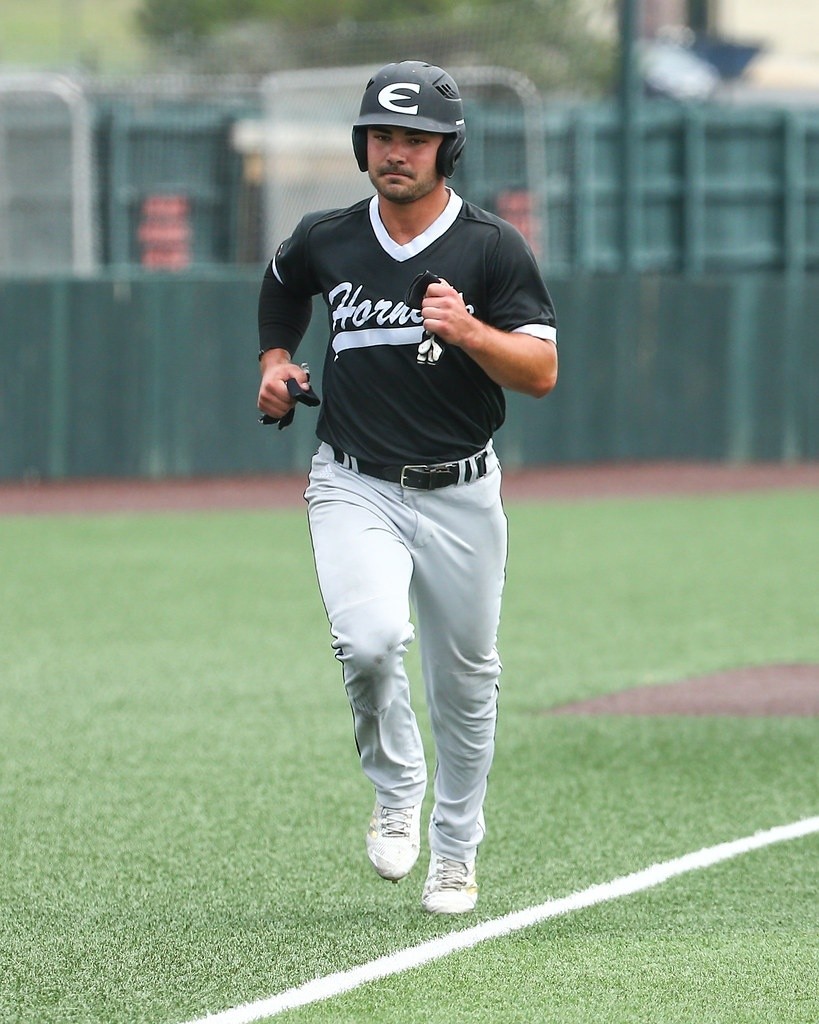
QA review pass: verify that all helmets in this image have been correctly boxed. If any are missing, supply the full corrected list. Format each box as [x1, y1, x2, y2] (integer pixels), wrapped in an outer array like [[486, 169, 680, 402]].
[[351, 60, 466, 179]]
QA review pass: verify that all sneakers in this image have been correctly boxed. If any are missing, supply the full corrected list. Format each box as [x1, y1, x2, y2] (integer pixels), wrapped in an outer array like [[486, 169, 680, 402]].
[[421, 852, 479, 914], [367, 800, 422, 882]]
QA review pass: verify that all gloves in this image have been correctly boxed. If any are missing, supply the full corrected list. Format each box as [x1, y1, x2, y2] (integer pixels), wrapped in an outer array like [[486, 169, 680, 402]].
[[259, 363, 322, 430], [406, 269, 447, 365]]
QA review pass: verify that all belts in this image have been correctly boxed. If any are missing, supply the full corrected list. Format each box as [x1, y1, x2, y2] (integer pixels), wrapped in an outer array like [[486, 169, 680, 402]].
[[333, 446, 486, 491]]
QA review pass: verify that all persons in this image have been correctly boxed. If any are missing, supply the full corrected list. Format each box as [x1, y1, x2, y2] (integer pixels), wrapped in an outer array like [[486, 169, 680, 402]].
[[259, 62, 558, 914]]
